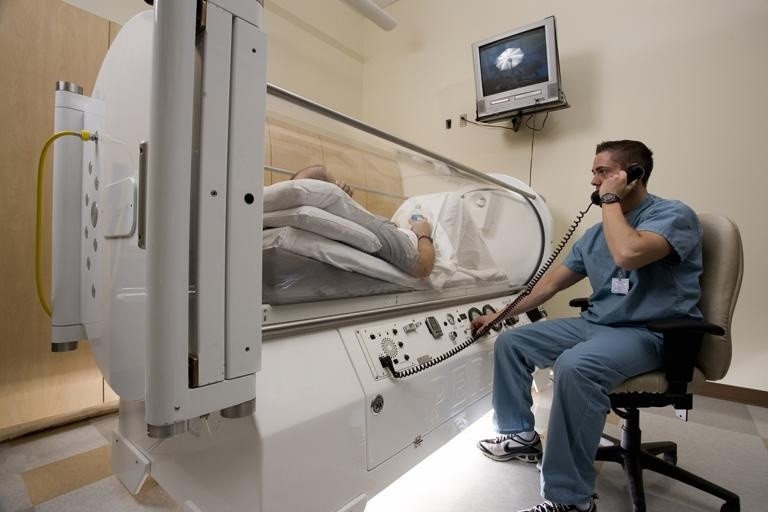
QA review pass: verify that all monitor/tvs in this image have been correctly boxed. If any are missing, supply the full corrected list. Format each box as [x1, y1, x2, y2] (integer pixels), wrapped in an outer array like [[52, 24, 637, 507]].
[[470, 15, 562, 120]]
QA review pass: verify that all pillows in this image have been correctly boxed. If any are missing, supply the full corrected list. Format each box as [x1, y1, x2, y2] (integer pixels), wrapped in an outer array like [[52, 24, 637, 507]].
[[262, 205, 383, 254], [261, 178, 375, 226]]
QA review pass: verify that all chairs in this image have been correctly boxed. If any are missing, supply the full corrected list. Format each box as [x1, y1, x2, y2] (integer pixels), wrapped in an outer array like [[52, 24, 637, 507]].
[[548, 211, 745, 511]]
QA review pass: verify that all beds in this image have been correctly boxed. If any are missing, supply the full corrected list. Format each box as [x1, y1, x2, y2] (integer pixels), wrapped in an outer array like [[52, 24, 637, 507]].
[[259, 227, 525, 331]]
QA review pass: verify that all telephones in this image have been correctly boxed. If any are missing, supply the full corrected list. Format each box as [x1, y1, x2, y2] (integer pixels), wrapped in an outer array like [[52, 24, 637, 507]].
[[591, 166, 644, 205]]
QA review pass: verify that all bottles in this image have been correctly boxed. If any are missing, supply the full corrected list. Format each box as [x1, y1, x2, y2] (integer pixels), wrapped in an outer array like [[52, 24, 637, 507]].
[[410, 202, 426, 227]]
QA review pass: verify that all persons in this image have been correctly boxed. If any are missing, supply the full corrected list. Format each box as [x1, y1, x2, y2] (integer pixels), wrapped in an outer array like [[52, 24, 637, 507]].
[[290, 165, 436, 278], [470, 140, 704, 512]]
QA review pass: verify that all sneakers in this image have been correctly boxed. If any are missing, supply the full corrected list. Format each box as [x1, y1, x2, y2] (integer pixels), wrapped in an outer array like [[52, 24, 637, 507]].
[[520, 500, 598, 511], [478, 432, 543, 463]]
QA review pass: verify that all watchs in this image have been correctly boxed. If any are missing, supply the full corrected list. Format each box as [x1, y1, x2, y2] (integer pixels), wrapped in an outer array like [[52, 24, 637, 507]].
[[599, 192, 624, 208]]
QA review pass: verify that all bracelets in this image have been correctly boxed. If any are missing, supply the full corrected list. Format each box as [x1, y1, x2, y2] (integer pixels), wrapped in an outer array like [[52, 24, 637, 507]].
[[418, 235, 433, 244]]
